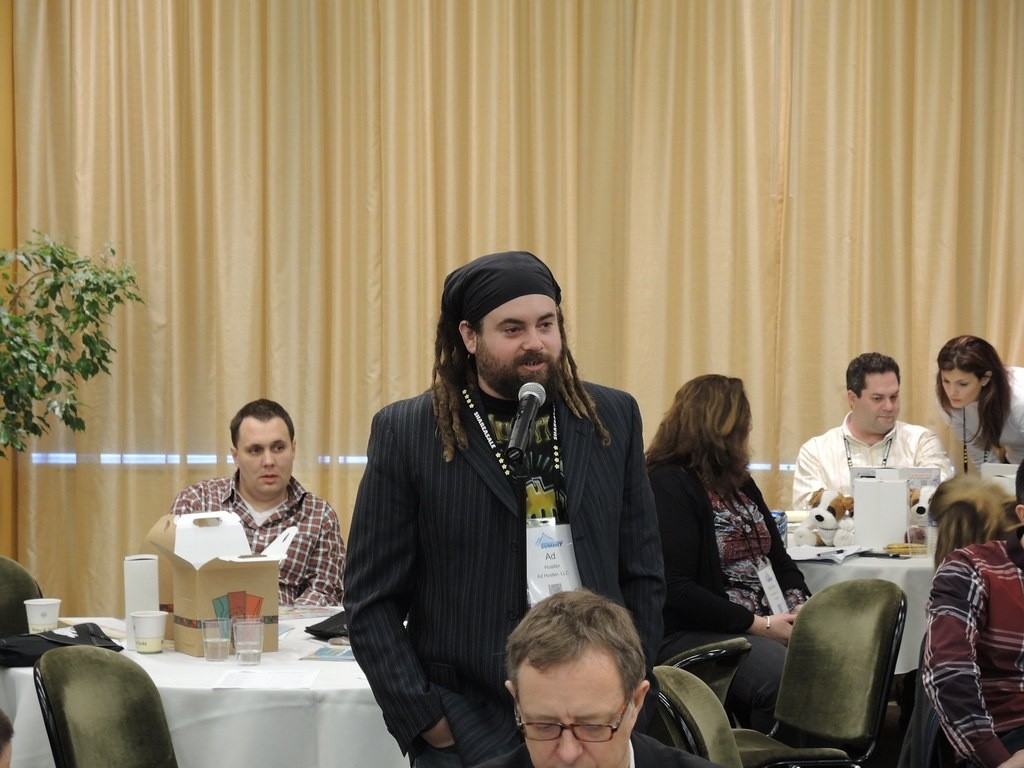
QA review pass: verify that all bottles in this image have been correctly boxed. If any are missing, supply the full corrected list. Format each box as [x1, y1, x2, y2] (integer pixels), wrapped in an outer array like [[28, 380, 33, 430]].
[[928, 514, 940, 561]]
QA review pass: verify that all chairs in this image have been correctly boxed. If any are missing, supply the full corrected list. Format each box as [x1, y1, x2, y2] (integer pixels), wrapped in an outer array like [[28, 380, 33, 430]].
[[653, 579, 908, 768], [34, 643, 178, 768]]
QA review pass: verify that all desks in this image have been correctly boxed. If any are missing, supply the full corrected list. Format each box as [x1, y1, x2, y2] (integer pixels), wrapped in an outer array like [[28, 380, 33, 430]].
[[795, 555, 934, 675], [0, 617, 410, 768]]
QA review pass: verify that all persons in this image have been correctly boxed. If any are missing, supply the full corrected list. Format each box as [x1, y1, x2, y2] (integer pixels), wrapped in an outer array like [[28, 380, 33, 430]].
[[899, 474, 1018, 733], [471, 590, 723, 768], [645, 373, 815, 749], [922, 460, 1024, 768], [0, 709, 14, 768], [792, 352, 952, 509], [343, 251, 666, 768], [934, 335, 1024, 469], [169, 399, 346, 606]]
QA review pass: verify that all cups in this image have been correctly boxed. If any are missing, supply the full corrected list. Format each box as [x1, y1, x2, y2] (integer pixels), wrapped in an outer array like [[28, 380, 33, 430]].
[[131, 611, 168, 653], [906, 509, 928, 557], [236, 614, 263, 650], [24, 598, 61, 634], [202, 618, 233, 661], [233, 621, 264, 665]]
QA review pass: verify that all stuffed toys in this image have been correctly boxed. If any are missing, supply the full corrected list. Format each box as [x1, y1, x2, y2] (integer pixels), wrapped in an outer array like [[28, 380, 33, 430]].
[[793, 490, 853, 547], [909, 486, 937, 528]]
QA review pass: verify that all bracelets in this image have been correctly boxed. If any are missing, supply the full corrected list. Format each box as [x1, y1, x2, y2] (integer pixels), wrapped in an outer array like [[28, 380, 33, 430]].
[[764, 616, 770, 628]]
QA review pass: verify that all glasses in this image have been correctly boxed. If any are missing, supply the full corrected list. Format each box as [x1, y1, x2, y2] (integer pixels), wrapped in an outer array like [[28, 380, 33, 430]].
[[513, 697, 629, 741]]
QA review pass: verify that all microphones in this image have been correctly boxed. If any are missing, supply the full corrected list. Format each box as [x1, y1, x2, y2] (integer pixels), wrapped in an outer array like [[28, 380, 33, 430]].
[[503, 381, 546, 463]]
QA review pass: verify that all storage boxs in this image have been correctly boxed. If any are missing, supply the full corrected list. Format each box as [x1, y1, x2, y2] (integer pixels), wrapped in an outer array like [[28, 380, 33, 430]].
[[149, 511, 298, 658]]
